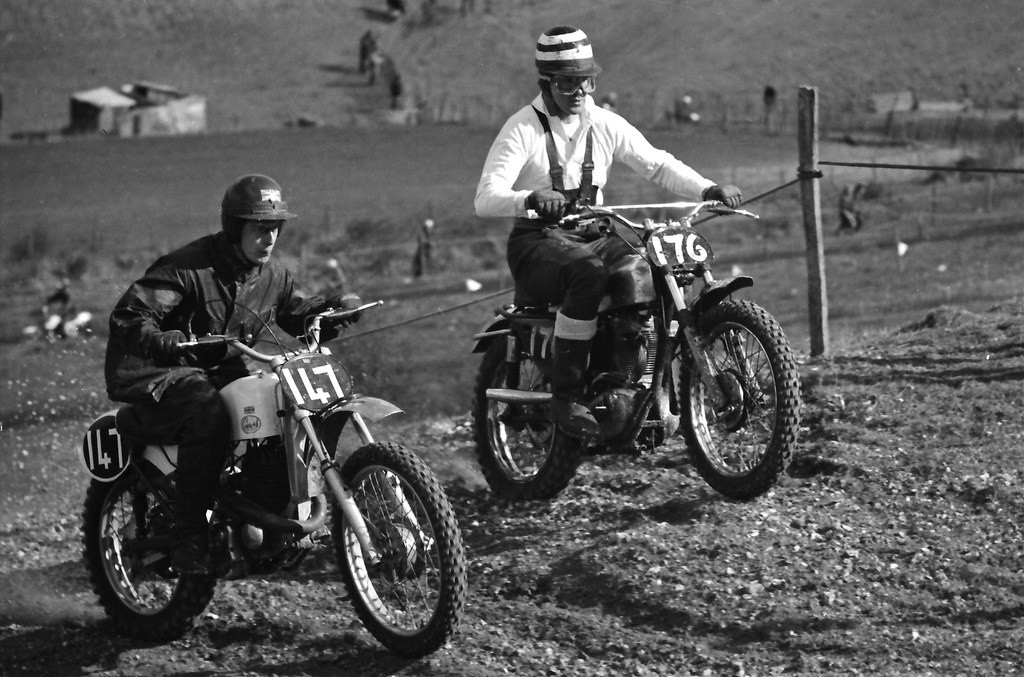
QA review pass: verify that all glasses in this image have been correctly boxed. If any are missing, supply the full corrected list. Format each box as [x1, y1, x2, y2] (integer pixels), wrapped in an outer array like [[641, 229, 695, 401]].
[[539, 77, 597, 95]]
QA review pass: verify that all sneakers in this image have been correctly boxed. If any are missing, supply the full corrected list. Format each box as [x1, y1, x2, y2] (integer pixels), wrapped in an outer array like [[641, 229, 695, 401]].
[[548, 380, 598, 431], [169, 503, 212, 574]]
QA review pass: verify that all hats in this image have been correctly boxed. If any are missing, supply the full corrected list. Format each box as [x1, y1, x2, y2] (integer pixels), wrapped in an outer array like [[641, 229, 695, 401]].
[[222, 174, 296, 221], [536, 26, 602, 78]]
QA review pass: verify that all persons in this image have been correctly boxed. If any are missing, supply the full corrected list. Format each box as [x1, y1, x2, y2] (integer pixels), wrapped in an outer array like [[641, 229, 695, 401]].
[[390, 75, 401, 108], [474, 27, 743, 438], [43, 279, 77, 339], [833, 185, 857, 234], [357, 29, 383, 84], [412, 219, 434, 277], [846, 182, 867, 231], [325, 259, 347, 295], [103, 174, 362, 579]]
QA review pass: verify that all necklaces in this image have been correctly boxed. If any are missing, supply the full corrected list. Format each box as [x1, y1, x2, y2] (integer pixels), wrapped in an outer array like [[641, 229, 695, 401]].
[[569, 137, 572, 141]]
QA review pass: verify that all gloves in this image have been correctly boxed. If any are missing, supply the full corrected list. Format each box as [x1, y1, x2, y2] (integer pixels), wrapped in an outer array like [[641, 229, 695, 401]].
[[526, 189, 566, 220], [320, 293, 363, 325], [704, 184, 743, 212], [140, 325, 189, 359]]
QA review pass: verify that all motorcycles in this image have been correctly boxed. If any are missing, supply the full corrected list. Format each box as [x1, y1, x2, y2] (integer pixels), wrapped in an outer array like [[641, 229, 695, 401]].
[[80, 299, 467, 660], [470, 197, 803, 500]]
